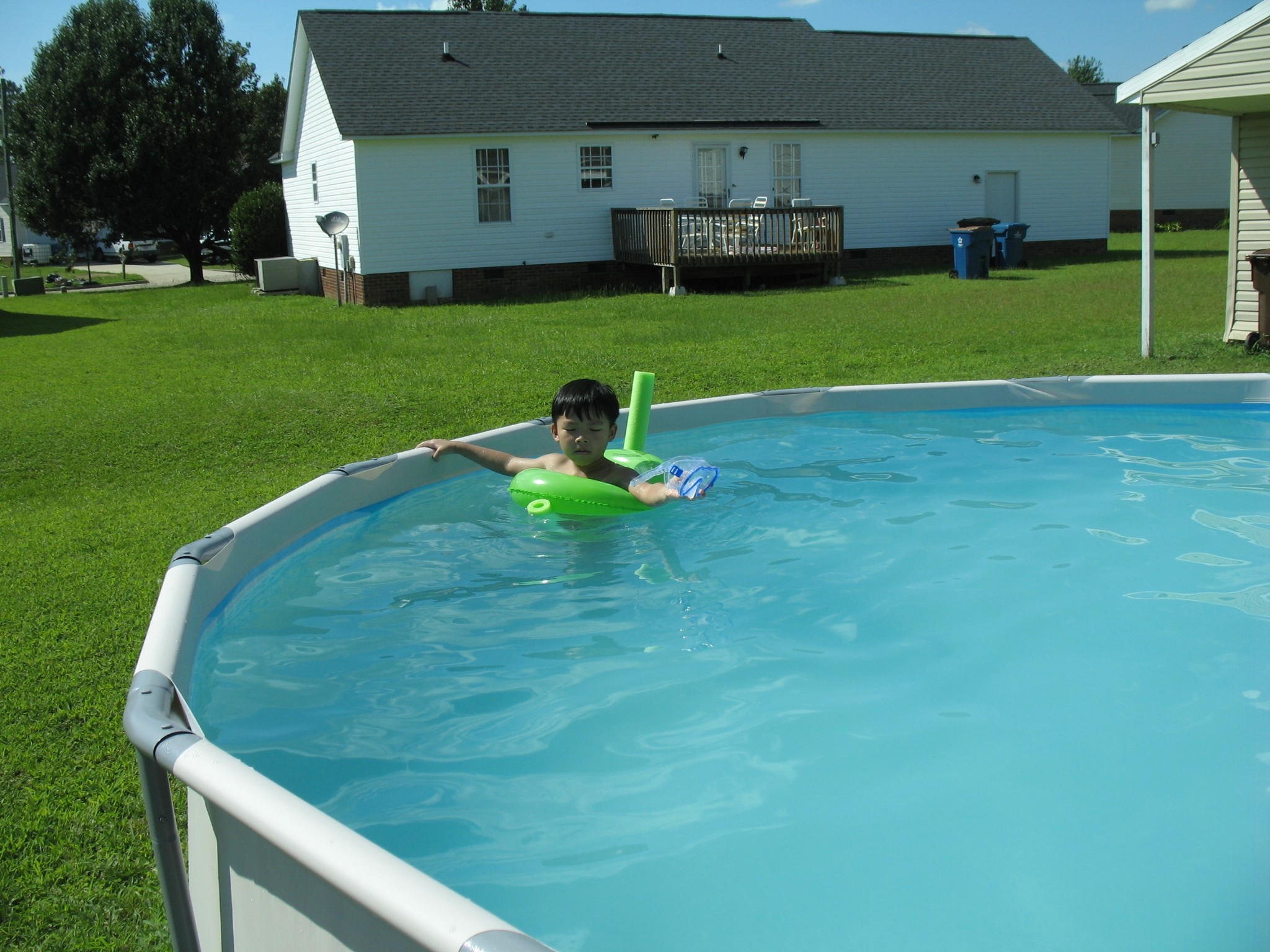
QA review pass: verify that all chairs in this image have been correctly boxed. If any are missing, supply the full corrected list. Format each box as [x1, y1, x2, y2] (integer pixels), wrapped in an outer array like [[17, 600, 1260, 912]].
[[658, 197, 767, 253], [791, 199, 827, 252]]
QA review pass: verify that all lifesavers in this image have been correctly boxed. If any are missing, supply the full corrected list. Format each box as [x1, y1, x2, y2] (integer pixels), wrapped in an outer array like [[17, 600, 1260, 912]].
[[509, 371, 663, 518]]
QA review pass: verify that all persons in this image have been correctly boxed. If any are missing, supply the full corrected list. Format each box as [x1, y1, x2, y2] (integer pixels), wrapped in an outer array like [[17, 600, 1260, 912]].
[[417, 378, 706, 507]]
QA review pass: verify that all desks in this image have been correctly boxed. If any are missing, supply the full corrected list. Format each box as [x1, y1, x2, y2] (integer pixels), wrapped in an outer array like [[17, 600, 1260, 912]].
[[681, 216, 733, 255]]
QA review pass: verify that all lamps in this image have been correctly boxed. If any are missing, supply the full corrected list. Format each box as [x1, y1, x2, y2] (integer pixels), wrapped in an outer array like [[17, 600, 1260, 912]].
[[739, 147, 749, 159]]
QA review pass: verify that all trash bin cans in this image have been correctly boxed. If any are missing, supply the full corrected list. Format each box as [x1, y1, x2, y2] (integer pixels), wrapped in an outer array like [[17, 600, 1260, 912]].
[[957, 217, 1001, 228], [948, 226, 994, 280], [991, 223, 1031, 268]]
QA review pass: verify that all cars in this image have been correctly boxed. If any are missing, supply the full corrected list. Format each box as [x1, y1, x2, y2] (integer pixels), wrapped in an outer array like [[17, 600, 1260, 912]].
[[96, 233, 160, 264], [199, 232, 233, 264]]
